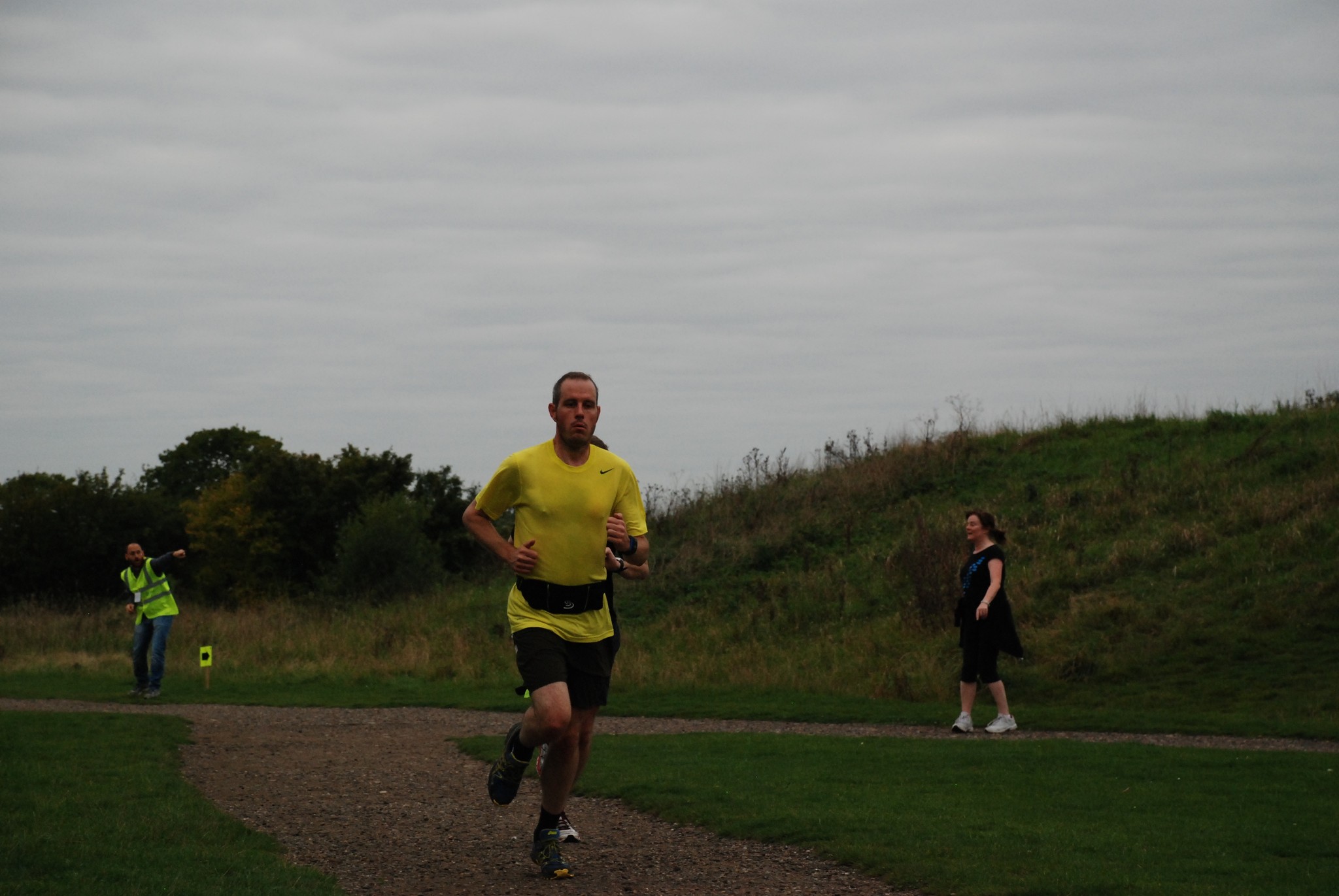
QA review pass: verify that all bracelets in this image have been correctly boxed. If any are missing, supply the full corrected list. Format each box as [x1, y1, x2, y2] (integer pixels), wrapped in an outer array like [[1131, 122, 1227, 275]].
[[980, 600, 990, 606], [623, 535, 638, 556], [615, 557, 628, 572]]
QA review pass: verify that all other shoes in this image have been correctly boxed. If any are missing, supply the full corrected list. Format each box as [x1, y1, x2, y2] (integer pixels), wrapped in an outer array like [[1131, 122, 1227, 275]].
[[132, 687, 144, 697], [144, 688, 161, 700]]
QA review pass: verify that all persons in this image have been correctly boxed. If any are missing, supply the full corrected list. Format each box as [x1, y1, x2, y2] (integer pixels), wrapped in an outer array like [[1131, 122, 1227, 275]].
[[119, 541, 186, 699], [950, 510, 1023, 733], [462, 371, 650, 881]]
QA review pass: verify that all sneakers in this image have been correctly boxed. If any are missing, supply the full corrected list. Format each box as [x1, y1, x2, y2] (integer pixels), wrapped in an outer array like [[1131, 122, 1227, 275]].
[[536, 742, 550, 778], [558, 812, 580, 842], [487, 723, 537, 807], [531, 827, 574, 879], [951, 713, 973, 733], [984, 714, 1018, 733]]
